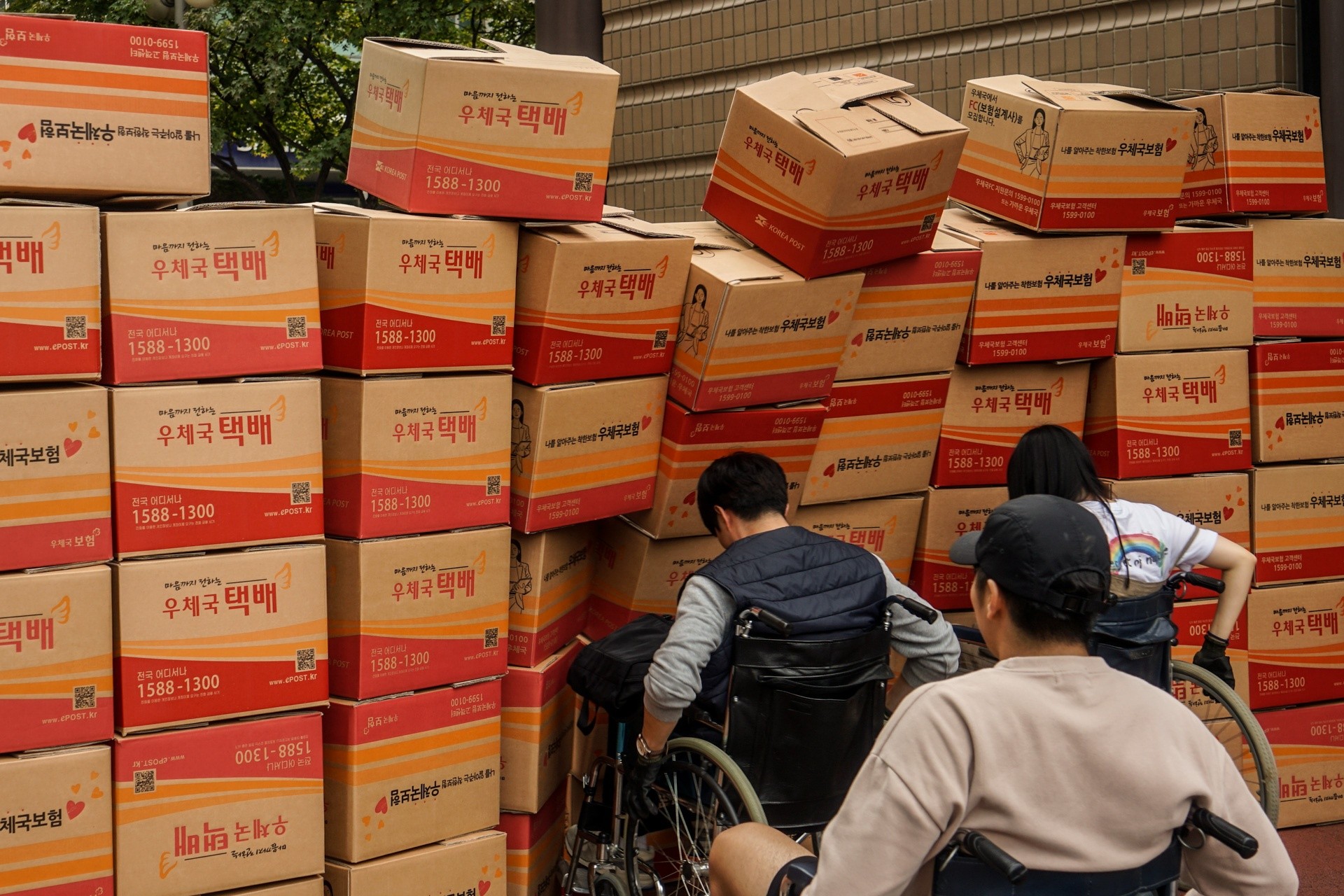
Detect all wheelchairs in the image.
[765,800,1256,896]
[950,569,1279,893]
[558,595,937,896]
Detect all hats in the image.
[949,493,1118,615]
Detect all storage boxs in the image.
[0,12,1344,896]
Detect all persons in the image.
[561,451,962,896]
[1005,425,1259,701]
[708,495,1300,896]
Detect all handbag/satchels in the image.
[565,612,732,737]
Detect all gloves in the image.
[1195,631,1234,704]
[621,757,662,824]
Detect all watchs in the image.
[636,734,670,758]
